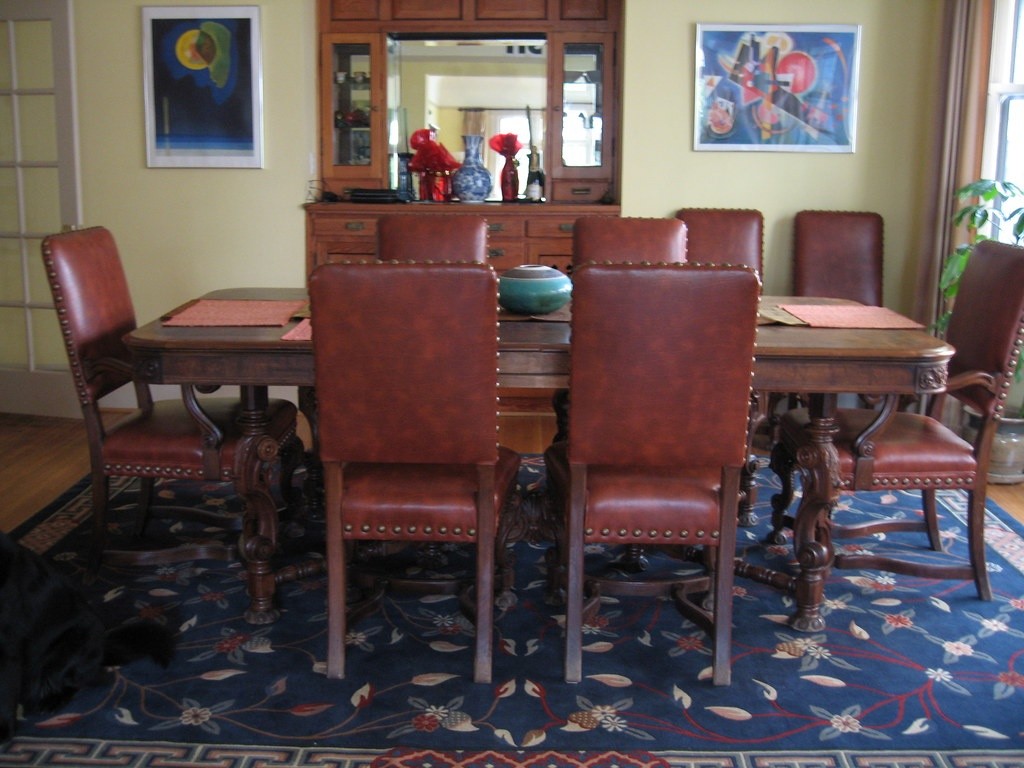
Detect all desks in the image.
[120,288,956,633]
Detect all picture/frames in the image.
[143,7,264,170]
[693,24,861,155]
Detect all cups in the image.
[355,71,364,84]
[336,71,347,83]
[419,170,451,202]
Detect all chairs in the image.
[39,208,1024,688]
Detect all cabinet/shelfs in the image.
[303,214,615,276]
[314,0,621,201]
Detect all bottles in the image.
[498,263,573,315]
[452,135,493,204]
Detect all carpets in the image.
[0,468,1024,768]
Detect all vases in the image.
[928,180,1024,484]
[452,135,492,201]
[501,154,519,201]
